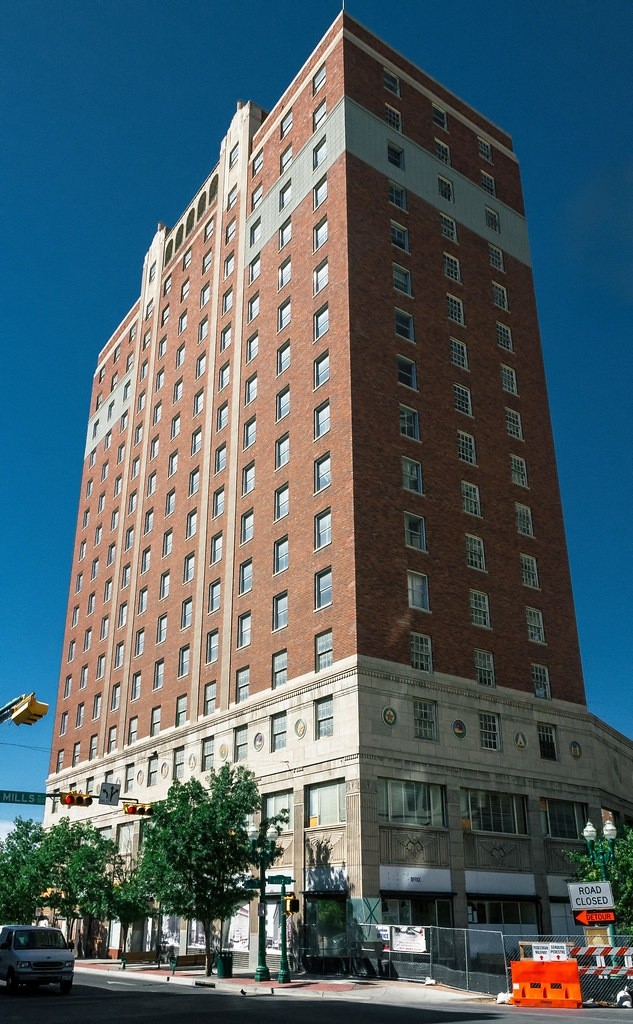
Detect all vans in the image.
[0,924,75,995]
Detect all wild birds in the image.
[240,988,247,997]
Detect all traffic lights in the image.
[10,697,50,726]
[60,793,93,807]
[123,804,156,817]
[287,899,299,913]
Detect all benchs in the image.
[120,951,163,970]
[171,953,216,976]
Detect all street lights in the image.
[584,819,617,965]
[254,824,278,981]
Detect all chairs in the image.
[9,936,21,946]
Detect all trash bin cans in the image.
[217,952,233,978]
